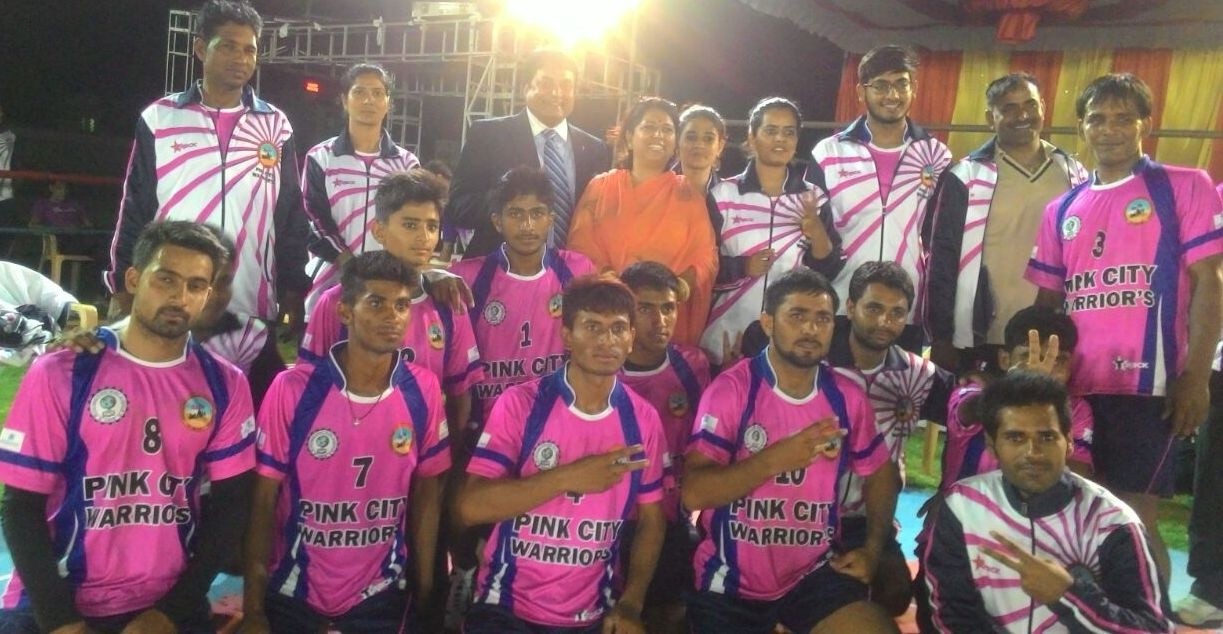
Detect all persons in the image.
[0,1,1223,634]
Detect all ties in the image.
[541,127,575,250]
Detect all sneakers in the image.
[444,563,480,633]
[1170,585,1223,624]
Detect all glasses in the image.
[864,79,913,92]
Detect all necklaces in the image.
[342,383,390,425]
